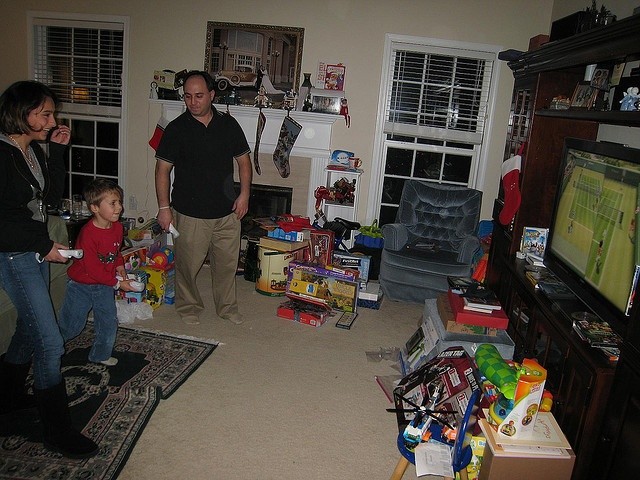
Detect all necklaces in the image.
[8,135,34,169]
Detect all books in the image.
[460,288,502,314]
[520,226,550,259]
[478,407,573,460]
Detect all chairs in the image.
[389,390,481,479]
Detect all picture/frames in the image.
[309,87,345,115]
[204,20,305,110]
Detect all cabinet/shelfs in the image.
[323,164,363,224]
[537,11,638,125]
[487,48,597,306]
[500,253,640,479]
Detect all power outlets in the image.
[129,195,137,210]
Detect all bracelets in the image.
[157,205,171,209]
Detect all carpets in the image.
[3,318,222,480]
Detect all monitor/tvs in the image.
[543,135,640,339]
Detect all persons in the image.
[256,64,264,94]
[154,69,253,326]
[261,67,286,95]
[1,81,100,461]
[59,178,137,366]
[340,98,351,128]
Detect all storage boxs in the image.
[474,396,577,480]
[421,298,516,359]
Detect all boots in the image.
[0,353,38,418]
[31,378,98,459]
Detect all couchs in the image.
[377,179,483,304]
[1,215,75,355]
